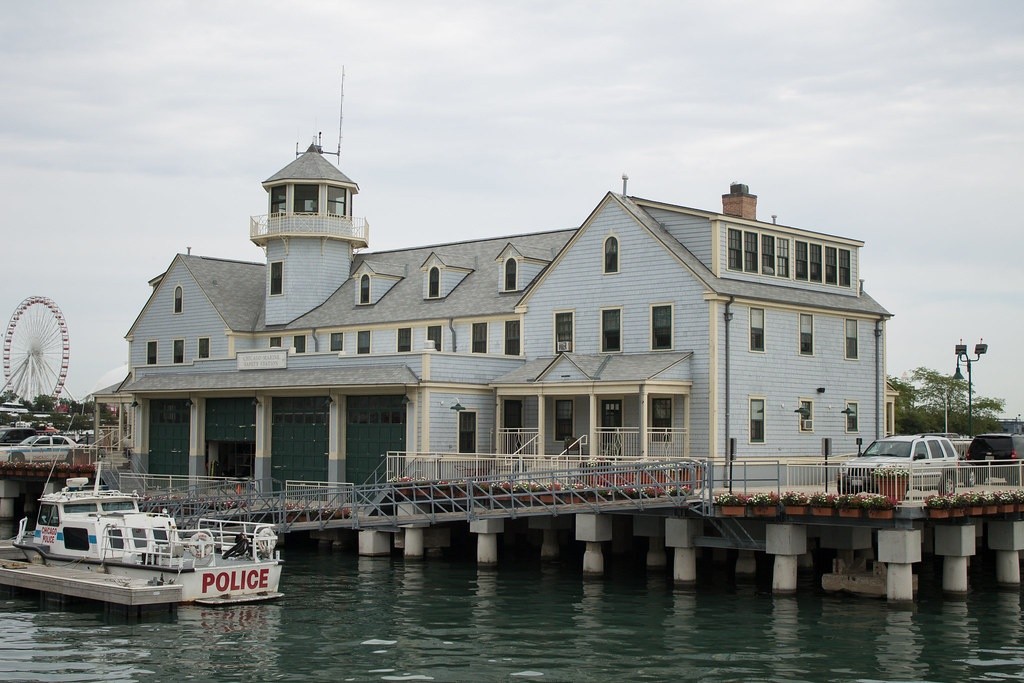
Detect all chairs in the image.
[564,436,584,461]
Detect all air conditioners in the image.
[557,341,572,351]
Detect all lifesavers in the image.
[257,527,278,553]
[188,532,215,558]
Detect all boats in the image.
[10,463,286,605]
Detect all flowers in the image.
[143,493,240,508]
[285,503,351,518]
[388,476,692,499]
[872,466,909,479]
[924,489,1024,509]
[716,491,896,512]
[0,461,96,474]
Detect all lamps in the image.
[780,404,810,414]
[817,388,825,393]
[325,397,333,404]
[251,397,259,406]
[828,405,855,414]
[440,397,466,414]
[129,401,138,407]
[186,399,194,406]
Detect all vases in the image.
[0,470,93,479]
[927,504,1024,518]
[154,507,194,514]
[722,507,893,520]
[397,490,597,504]
[876,476,908,501]
[285,514,307,522]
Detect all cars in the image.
[955,450,977,487]
[0,435,90,465]
[0,427,38,447]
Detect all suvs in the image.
[836,434,960,501]
[965,432,1024,486]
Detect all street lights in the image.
[951,338,988,440]
